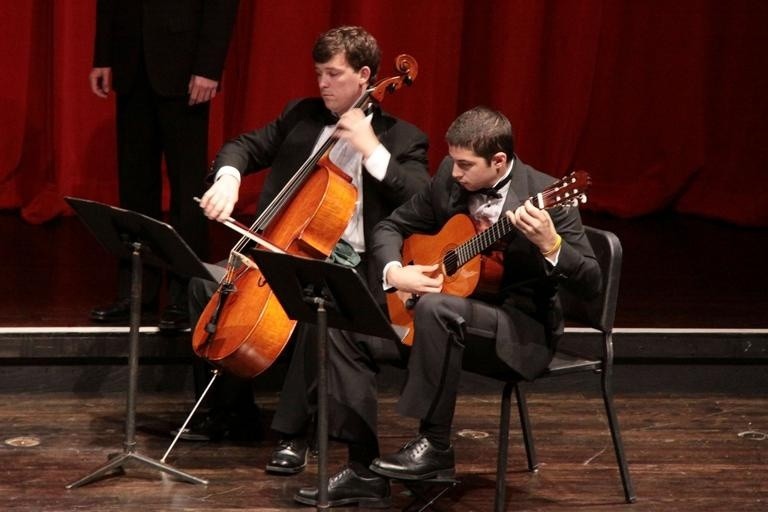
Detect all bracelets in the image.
[540,235,563,258]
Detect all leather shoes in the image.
[158,300,191,328]
[369,437,459,483]
[292,469,393,510]
[263,437,314,473]
[169,407,263,441]
[86,291,149,324]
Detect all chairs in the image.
[448,226,637,512]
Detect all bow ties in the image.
[472,154,517,200]
[321,105,377,126]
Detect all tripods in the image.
[63,244,210,490]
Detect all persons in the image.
[294,104,606,509]
[90,0,240,329]
[169,25,429,475]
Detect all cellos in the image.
[191,53,419,380]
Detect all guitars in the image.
[385,168,592,348]
